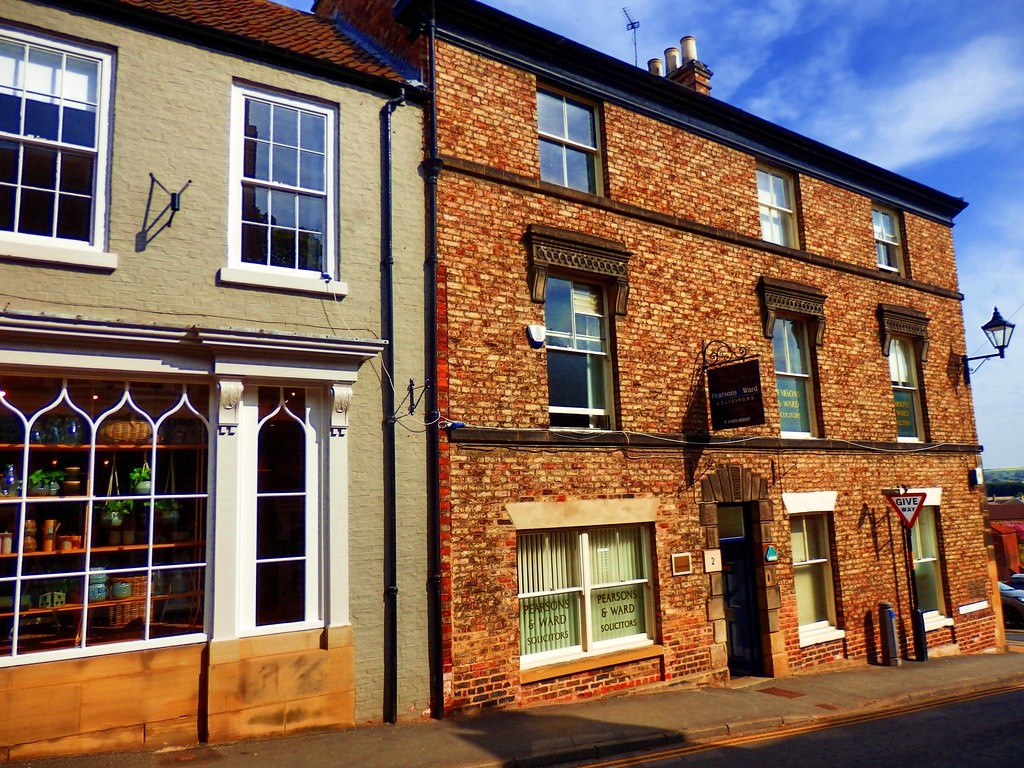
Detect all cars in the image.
[998,581,1024,627]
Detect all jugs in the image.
[30,417,83,444]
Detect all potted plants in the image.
[27,470,68,497]
[92,500,135,528]
[144,500,180,523]
[128,467,151,494]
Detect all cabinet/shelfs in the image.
[0,421,208,657]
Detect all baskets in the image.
[96,418,165,446]
[97,575,154,628]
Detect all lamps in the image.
[150,173,191,227]
[960,306,1016,384]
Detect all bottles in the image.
[89,567,132,603]
[154,562,166,596]
[108,515,136,545]
[171,562,190,593]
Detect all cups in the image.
[0,518,81,554]
